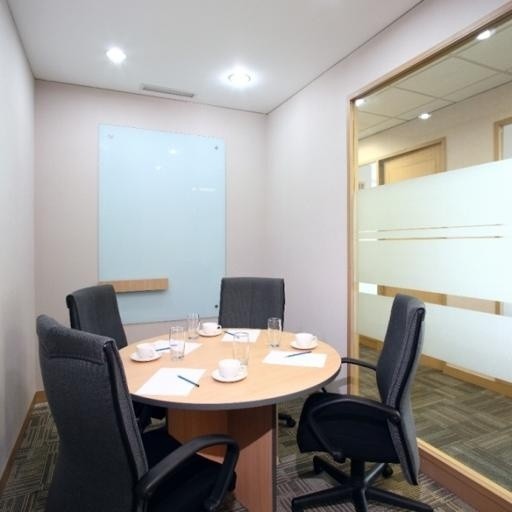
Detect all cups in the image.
[202,321,222,334]
[267,316,281,348]
[295,333,317,347]
[232,332,249,366]
[136,342,156,359]
[186,310,201,341]
[218,359,246,379]
[169,326,186,362]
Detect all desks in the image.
[117,327,341,511]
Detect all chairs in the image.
[292,294,436,512]
[219,277,285,329]
[37,315,240,512]
[66,285,127,351]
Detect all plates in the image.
[130,350,161,362]
[290,340,317,350]
[199,329,220,337]
[212,364,248,383]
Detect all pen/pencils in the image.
[177,376,199,387]
[224,330,234,336]
[288,350,312,357]
[156,347,170,352]
